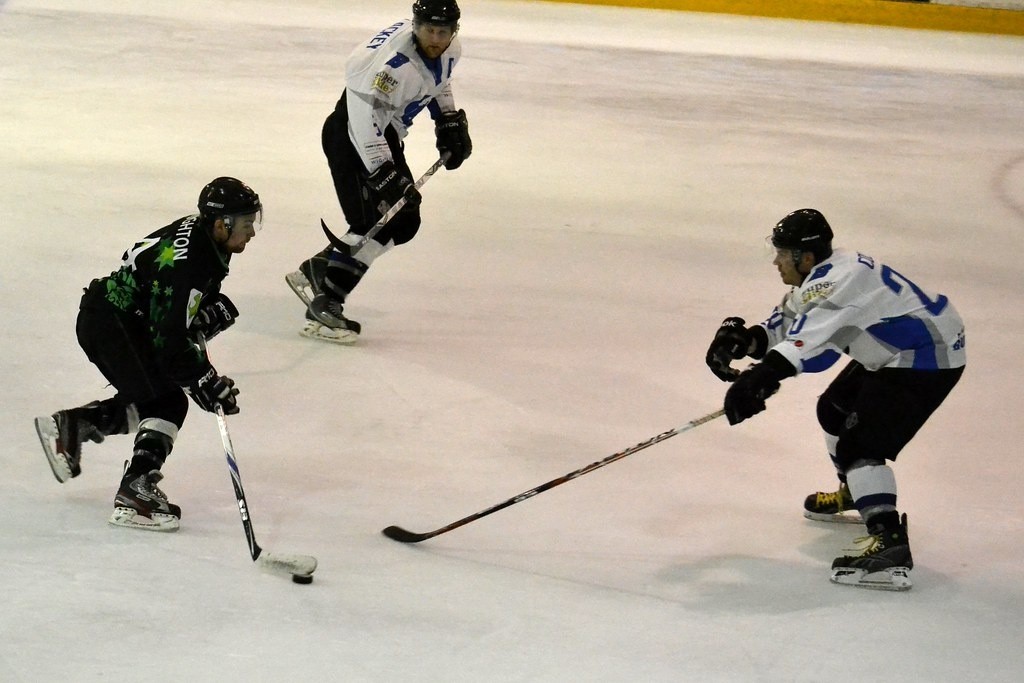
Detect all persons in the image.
[286,0,473,343]
[35,176,262,531]
[706,209,967,592]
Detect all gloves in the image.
[186,359,240,416]
[367,161,421,226]
[706,317,753,382]
[435,109,472,170]
[724,350,798,426]
[188,293,239,344]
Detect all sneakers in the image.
[299,292,361,346]
[285,255,336,308]
[830,512,914,592]
[108,459,182,533]
[803,472,866,525]
[34,408,105,484]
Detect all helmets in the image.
[412,0,460,27]
[197,176,262,218]
[771,209,833,250]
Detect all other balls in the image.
[292,573,312,585]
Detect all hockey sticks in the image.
[198,331,317,578]
[314,151,453,257]
[382,409,724,549]
